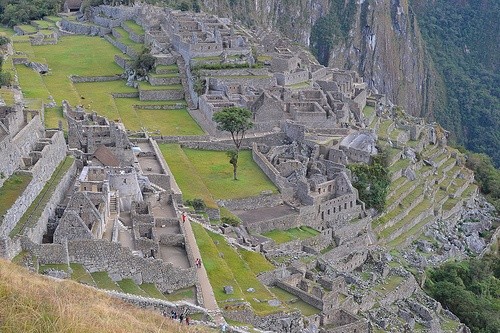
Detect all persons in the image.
[183,215,186,223]
[163,309,191,325]
[198,259,202,267]
[196,258,199,267]
